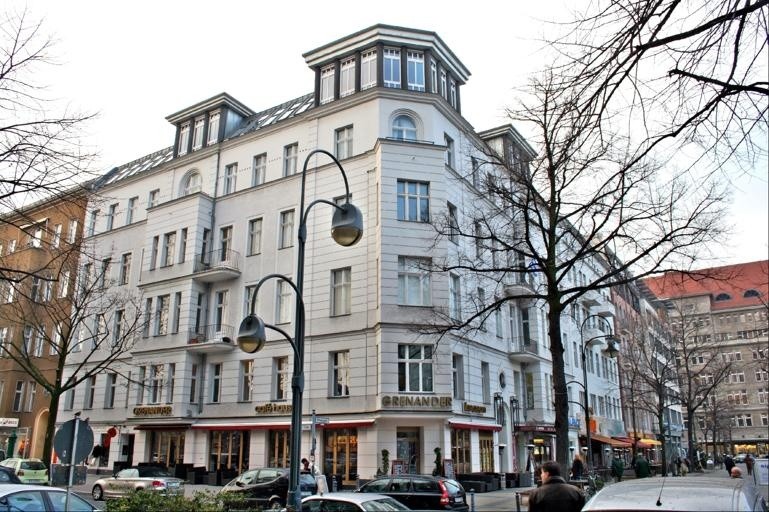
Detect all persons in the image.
[725,455,735,476]
[528,462,586,512]
[674,456,682,475]
[631,452,652,478]
[572,453,584,479]
[615,458,624,482]
[745,454,753,475]
[301,458,321,475]
[682,458,692,473]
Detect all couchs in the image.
[456,472,531,492]
[113,461,238,486]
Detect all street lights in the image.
[234,148,366,511]
[581,314,620,494]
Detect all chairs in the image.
[336,474,356,491]
